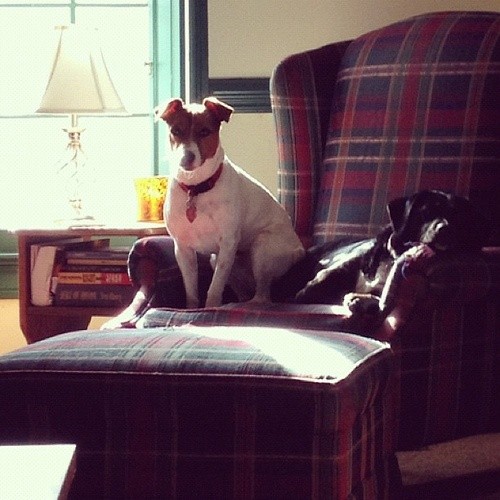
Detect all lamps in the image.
[36,26,127,223]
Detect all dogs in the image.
[151,95,306,310]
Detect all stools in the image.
[0,324,395,499]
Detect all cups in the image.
[137,177,168,222]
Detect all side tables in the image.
[15,226,169,344]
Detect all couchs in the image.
[101,11,500,453]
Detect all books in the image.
[52,249,133,307]
